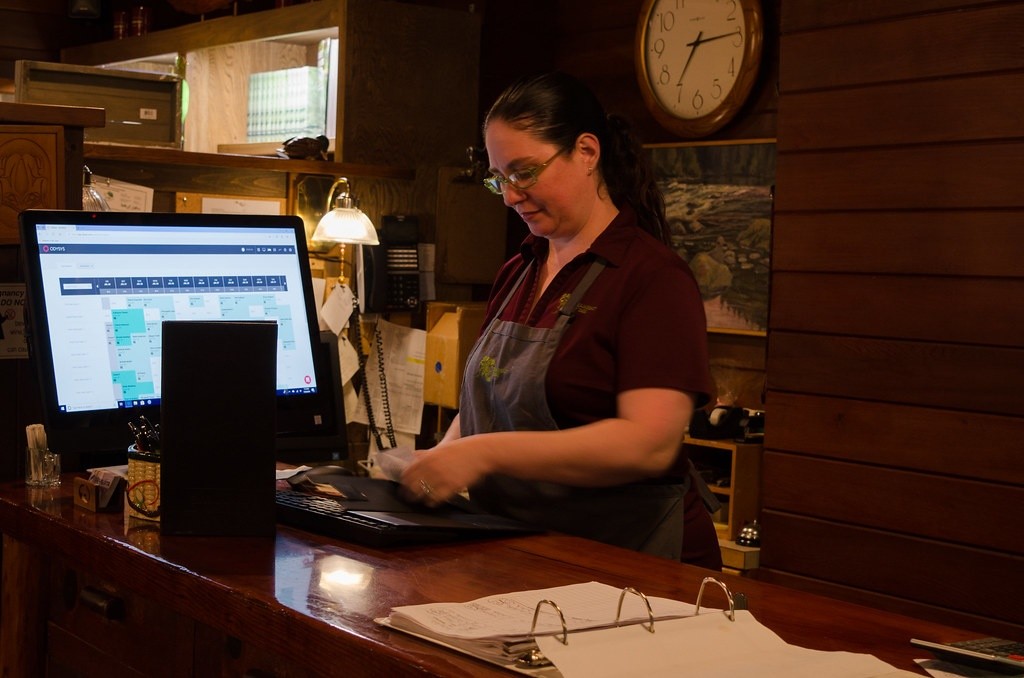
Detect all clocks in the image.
[634,0,765,142]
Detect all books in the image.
[371,580,940,678]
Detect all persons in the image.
[378,70,724,572]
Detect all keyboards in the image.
[275,489,422,549]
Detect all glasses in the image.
[481,146,566,194]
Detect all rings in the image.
[418,480,434,495]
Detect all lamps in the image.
[311,177,382,248]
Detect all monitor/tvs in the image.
[20,208,335,455]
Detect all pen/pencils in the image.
[127,413,160,452]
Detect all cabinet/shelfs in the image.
[681,435,763,540]
